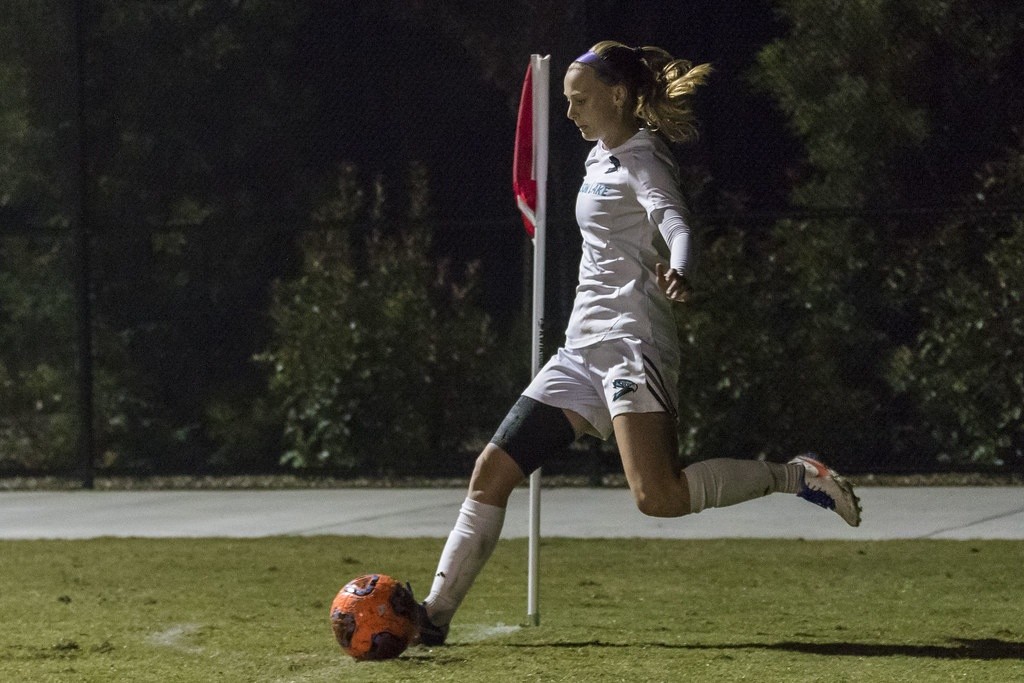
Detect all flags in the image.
[513,63,536,240]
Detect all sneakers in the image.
[406,579,448,644]
[789,451,863,529]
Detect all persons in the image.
[415,41,863,646]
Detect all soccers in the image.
[329,573,413,661]
